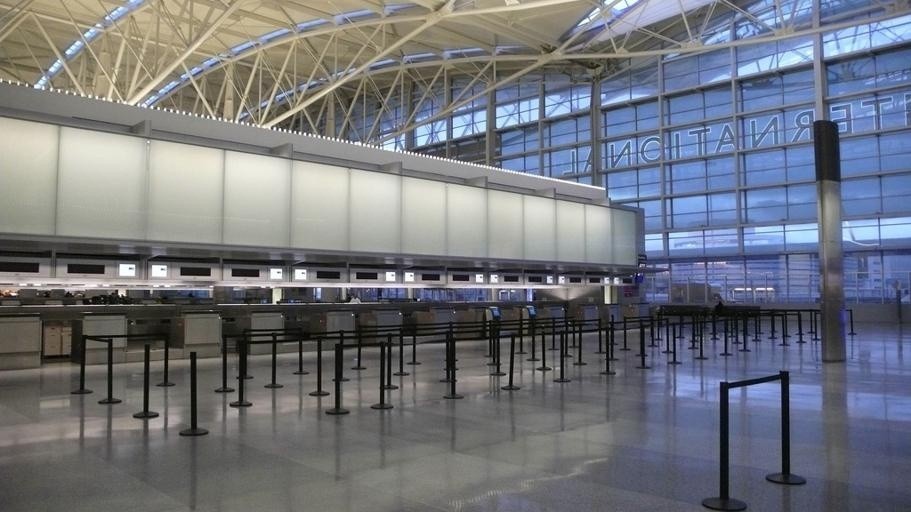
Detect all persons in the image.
[713,296,724,307]
[349,292,361,303]
